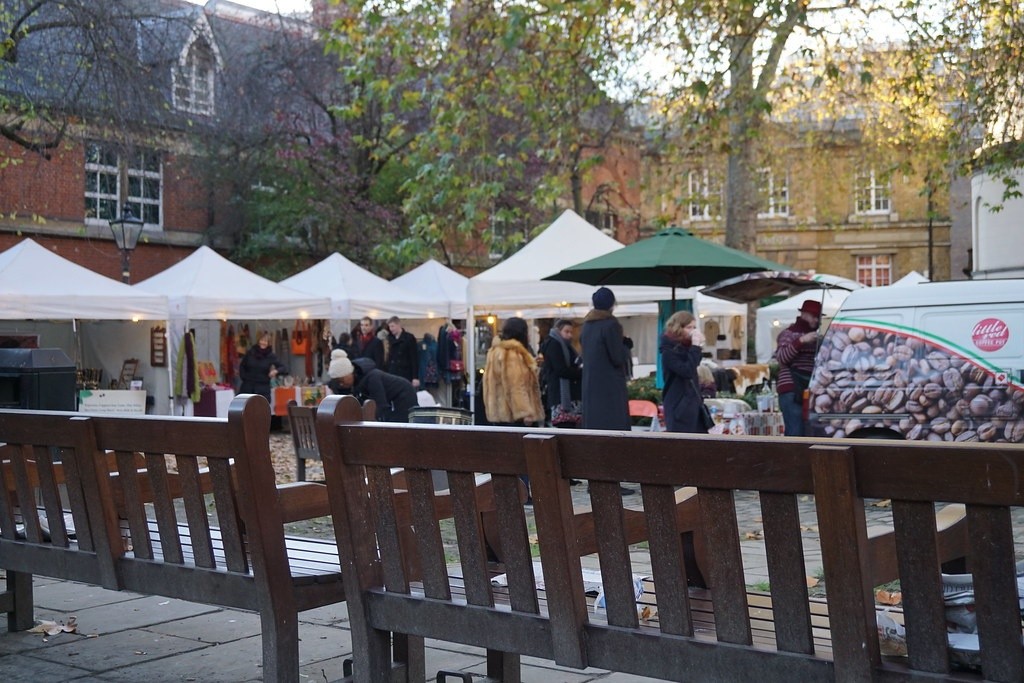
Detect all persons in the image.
[238,287,826,504]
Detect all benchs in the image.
[0,394,424,683]
[317,397,1024,683]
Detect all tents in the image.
[0,208,931,425]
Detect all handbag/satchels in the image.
[701,402,715,431]
[790,367,812,405]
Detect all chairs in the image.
[629,400,660,432]
[286,400,376,481]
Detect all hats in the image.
[327,349,354,378]
[592,287,615,309]
[798,300,827,316]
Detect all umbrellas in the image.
[540,226,800,314]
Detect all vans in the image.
[808,276,1024,443]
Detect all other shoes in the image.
[523,495,534,505]
[620,486,635,495]
[570,480,582,486]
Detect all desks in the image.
[709,411,788,436]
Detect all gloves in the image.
[623,336,634,349]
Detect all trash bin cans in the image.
[406,405,474,491]
[0,347,78,463]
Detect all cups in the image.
[689,329,705,346]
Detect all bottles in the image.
[762,381,770,412]
[771,381,779,411]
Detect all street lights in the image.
[108,201,144,287]
